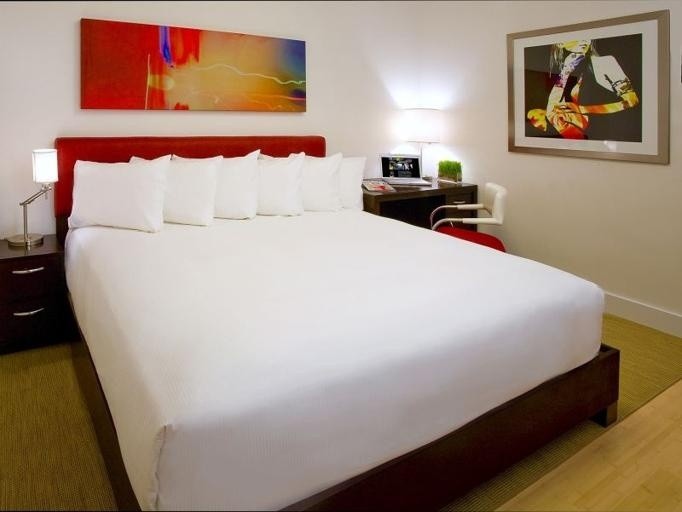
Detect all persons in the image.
[526,39,639,139]
[402,163,406,171]
[393,160,399,177]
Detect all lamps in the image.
[5,148,59,249]
[401,108,446,181]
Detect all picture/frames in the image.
[504,7,670,167]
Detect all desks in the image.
[359,172,477,229]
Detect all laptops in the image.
[381,155,432,185]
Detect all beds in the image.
[52,135,619,511]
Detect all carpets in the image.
[1,308,682,511]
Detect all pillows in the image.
[290,153,341,211]
[68,156,170,233]
[173,151,259,222]
[130,154,222,227]
[253,151,306,217]
[331,156,368,210]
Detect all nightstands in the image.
[1,233,77,355]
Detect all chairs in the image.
[428,183,509,254]
